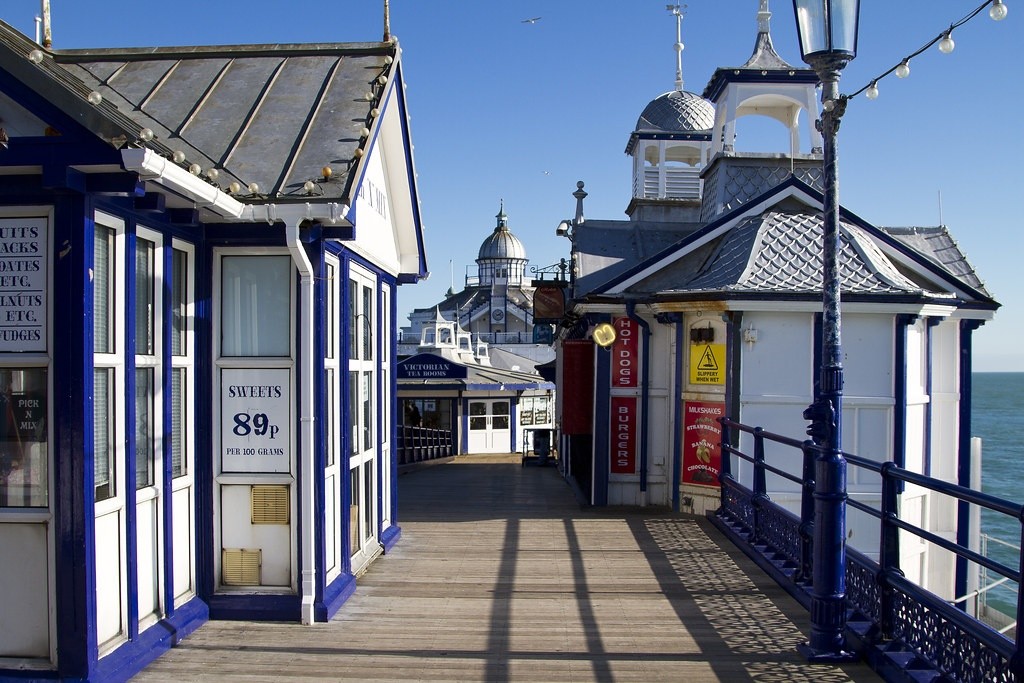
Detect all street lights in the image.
[791,0,861,664]
